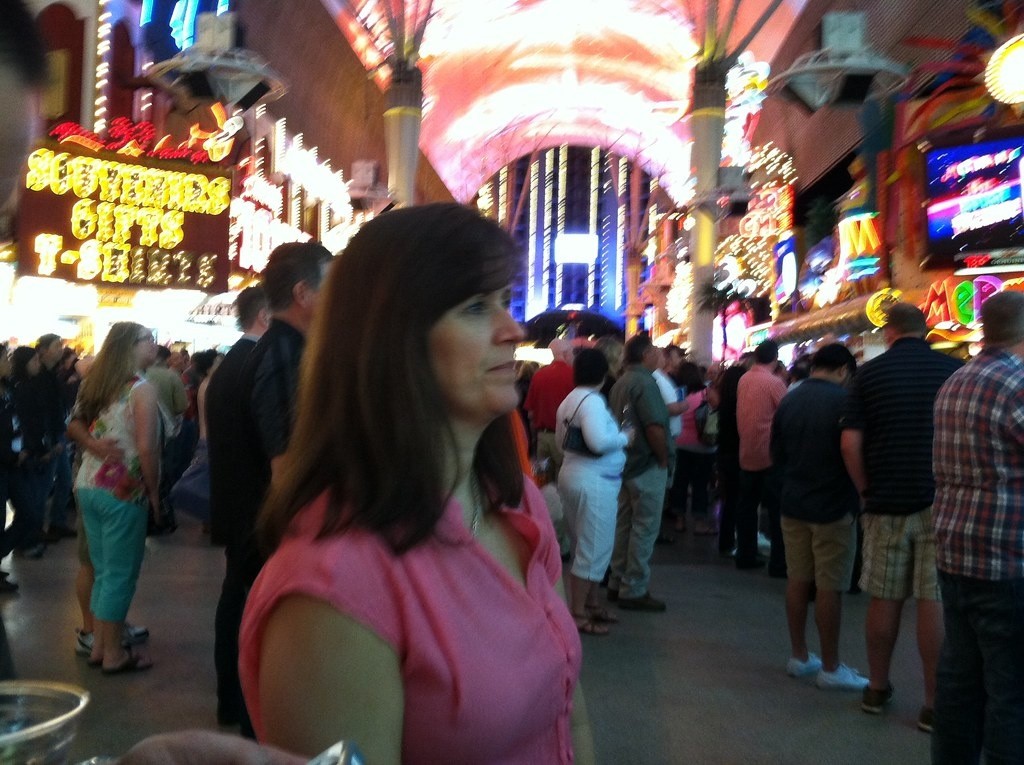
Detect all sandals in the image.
[576,606,616,635]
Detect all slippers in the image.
[675,526,687,532]
[693,527,719,536]
[86,653,153,675]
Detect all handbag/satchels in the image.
[168,439,210,521]
[694,403,718,446]
[560,392,608,458]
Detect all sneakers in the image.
[816,663,869,691]
[861,681,895,713]
[918,705,933,732]
[787,652,822,677]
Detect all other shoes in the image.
[606,588,666,612]
[737,559,765,570]
[77,626,150,650]
[14,545,46,559]
[768,565,787,578]
[0,572,19,593]
[47,524,78,539]
[719,542,738,557]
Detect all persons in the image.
[838,303,965,732]
[233,243,334,743]
[237,201,593,764]
[517,330,814,635]
[0,0,55,765]
[929,292,1024,765]
[205,284,272,742]
[64,322,159,674]
[0,333,225,655]
[770,345,869,690]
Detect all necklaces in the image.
[472,475,481,538]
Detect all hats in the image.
[15,346,37,365]
